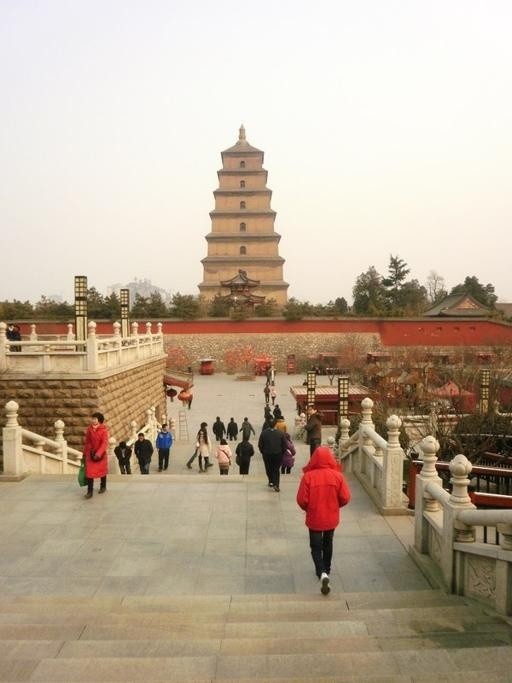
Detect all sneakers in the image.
[320,573,330,595]
[86,492,92,498]
[269,482,280,491]
[99,487,106,493]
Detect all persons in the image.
[5,324,14,352]
[12,326,21,352]
[83,412,109,500]
[115,366,324,492]
[388,384,414,410]
[296,444,352,596]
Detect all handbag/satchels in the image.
[78,459,88,486]
[90,448,104,461]
